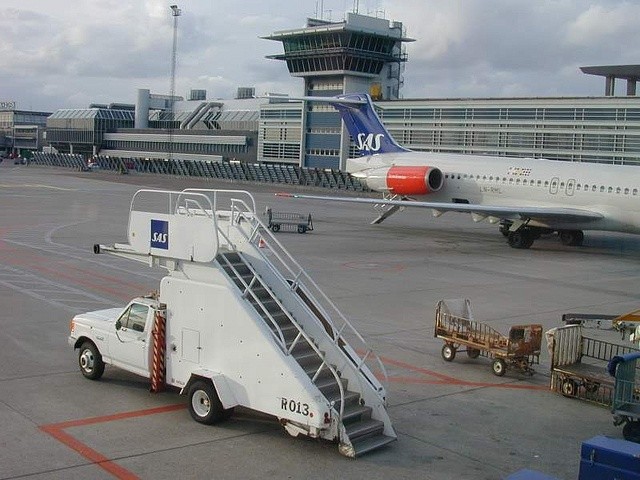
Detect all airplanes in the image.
[252,90,640,250]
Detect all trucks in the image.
[434,297,544,377]
[610,347,640,443]
[263,205,314,234]
[548,323,640,411]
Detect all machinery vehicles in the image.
[66,185,401,460]
